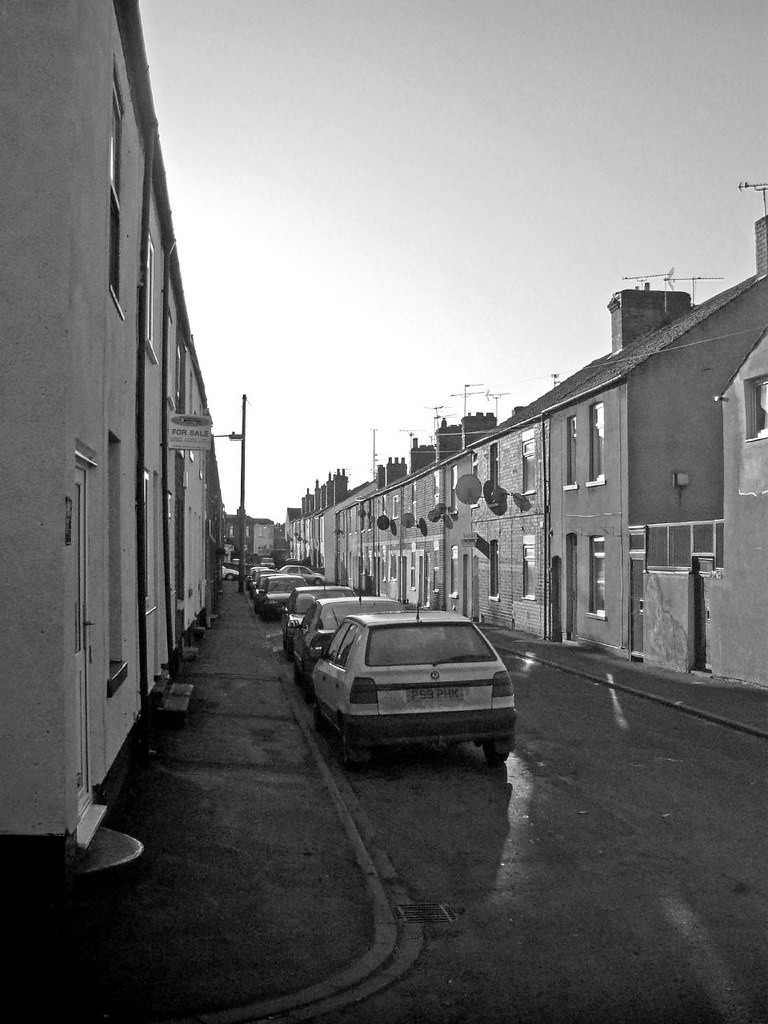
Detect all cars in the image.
[244,567,289,599]
[280,586,357,660]
[232,559,240,564]
[311,611,516,768]
[279,565,327,586]
[254,576,308,620]
[286,596,407,701]
[261,558,275,569]
[222,566,239,581]
[285,558,302,564]
